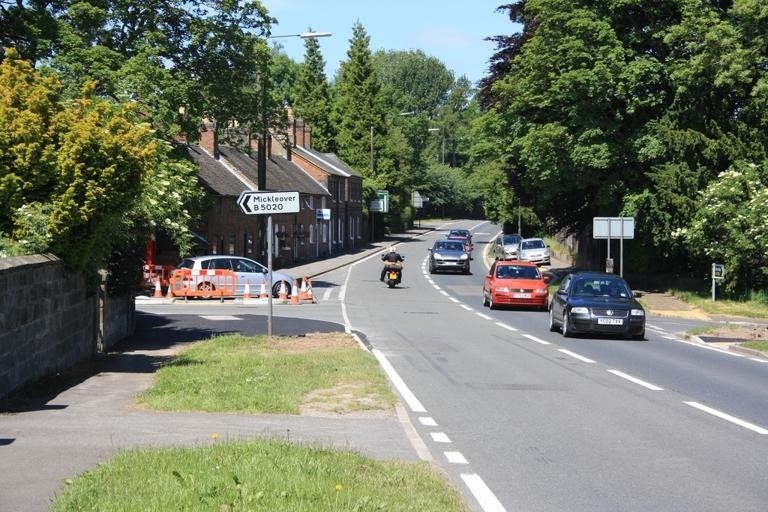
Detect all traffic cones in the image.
[164,280,175,300]
[241,272,315,305]
[152,275,163,298]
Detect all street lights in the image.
[255,25,333,267]
[368,110,414,177]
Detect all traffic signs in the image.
[234,190,303,215]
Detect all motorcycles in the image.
[379,253,406,287]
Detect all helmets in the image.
[389,245,396,253]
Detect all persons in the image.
[380,246,404,281]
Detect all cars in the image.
[168,255,297,299]
[426,239,471,275]
[479,259,553,313]
[545,270,649,345]
[493,233,525,262]
[445,226,473,262]
[514,238,553,267]
